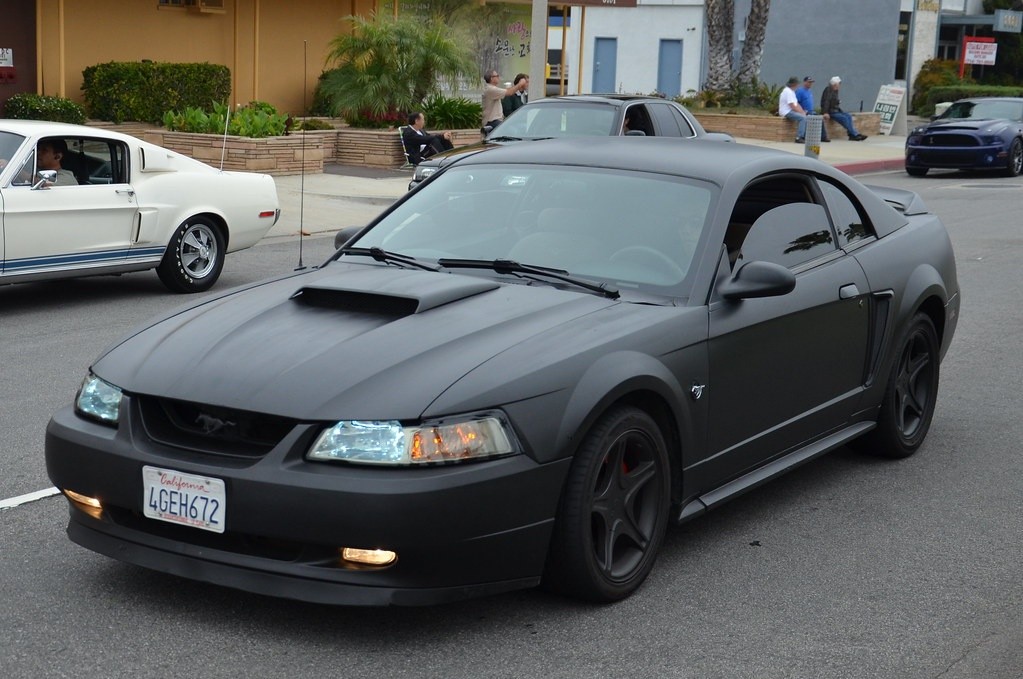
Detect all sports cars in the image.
[904,97,1023,177]
[46,137,963,610]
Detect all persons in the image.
[796,75,831,143]
[821,76,868,141]
[0,136,79,186]
[483,68,527,132]
[502,73,529,117]
[402,112,454,165]
[778,77,807,143]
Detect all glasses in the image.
[492,75,499,78]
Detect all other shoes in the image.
[796,137,804,143]
[849,135,862,141]
[857,133,868,141]
[822,138,831,143]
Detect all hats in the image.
[803,76,815,83]
[785,77,801,86]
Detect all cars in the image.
[0,120,282,294]
[405,93,736,196]
